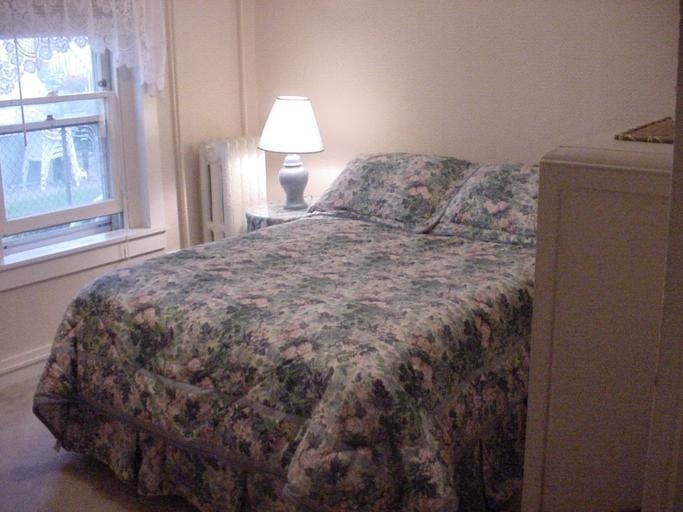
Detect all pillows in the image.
[308,150,540,245]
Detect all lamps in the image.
[256,95,325,210]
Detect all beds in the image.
[65,211,536,511]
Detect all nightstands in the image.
[244,205,311,235]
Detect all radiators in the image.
[196,134,266,245]
